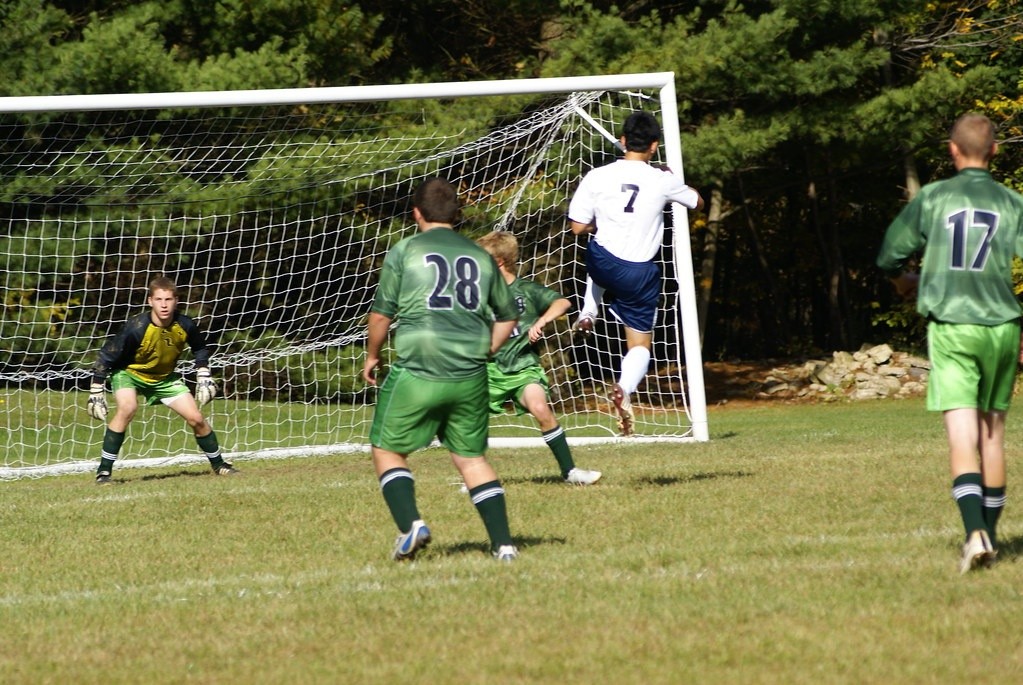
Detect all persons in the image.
[878,113,1023,576]
[362,178,520,562]
[87,278,241,486]
[568,111,704,436]
[473,231,602,486]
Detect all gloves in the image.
[86,383,113,421]
[194,367,219,406]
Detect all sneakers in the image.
[95,470,112,483]
[214,460,240,476]
[959,528,998,576]
[563,467,602,487]
[391,519,433,562]
[571,312,596,340]
[607,383,636,436]
[490,544,520,562]
